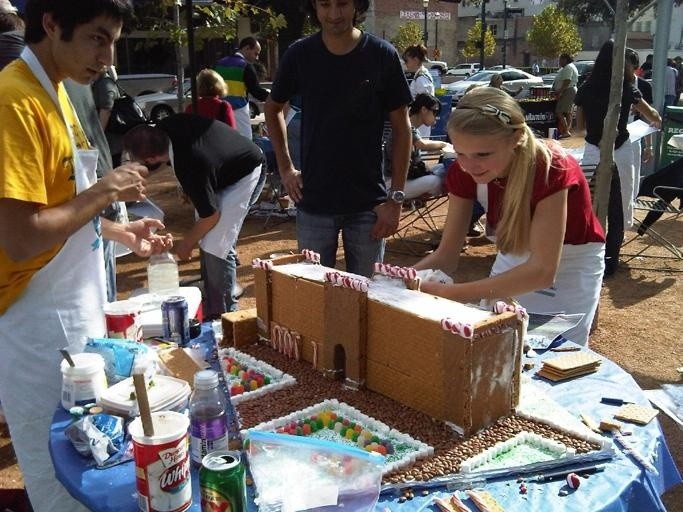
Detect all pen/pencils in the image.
[152,338,170,345]
[551,337,581,352]
[537,465,606,482]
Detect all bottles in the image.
[147,244,179,302]
[189,370,228,469]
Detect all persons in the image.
[1,1,272,510]
[412,87,606,349]
[552,39,683,277]
[264,1,414,276]
[383,44,538,238]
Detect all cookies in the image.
[536,351,602,382]
[600,421,621,431]
[580,413,603,434]
[432,491,506,512]
[615,404,659,425]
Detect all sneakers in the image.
[559,132,571,138]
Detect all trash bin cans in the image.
[430,96,451,141]
[660,105,683,170]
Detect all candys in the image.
[251,410,394,474]
[218,355,271,396]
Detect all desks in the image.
[47,299,682,512]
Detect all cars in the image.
[398,53,448,78]
[446,62,484,78]
[442,67,543,106]
[541,60,595,87]
[134,77,271,124]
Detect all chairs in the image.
[614,185,682,269]
[413,137,479,230]
[380,141,449,253]
[252,111,307,230]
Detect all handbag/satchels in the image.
[96,76,148,140]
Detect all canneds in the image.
[161,296,190,347]
[199,450,248,512]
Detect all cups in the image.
[103,300,144,343]
[128,411,192,512]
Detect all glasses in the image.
[427,107,440,117]
